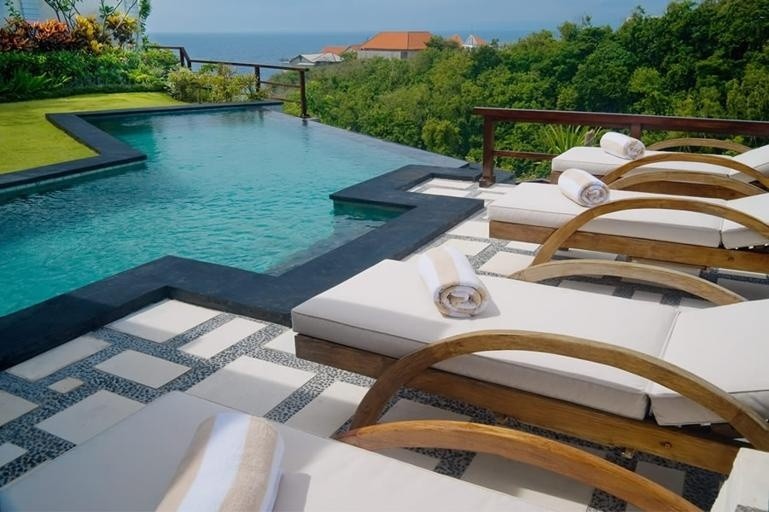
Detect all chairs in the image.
[474,170,769,308]
[285,248,769,486]
[0,384,769,512]
[546,140,768,200]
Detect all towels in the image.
[600,131,645,160]
